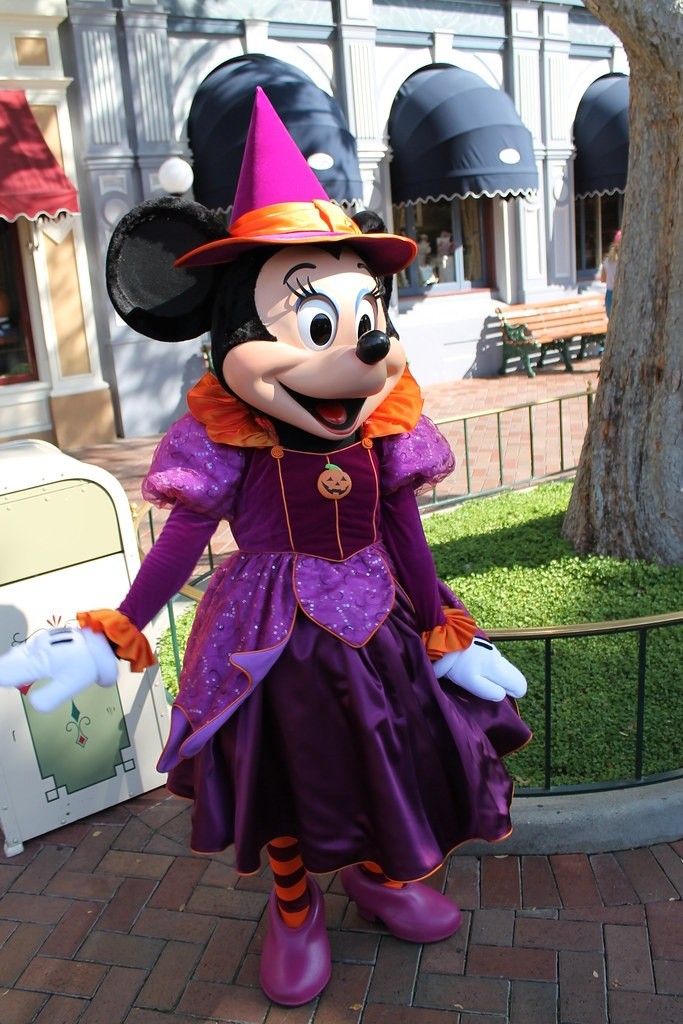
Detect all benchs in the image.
[495,294,608,379]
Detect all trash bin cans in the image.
[0,437,166,858]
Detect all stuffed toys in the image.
[0,87,532,1006]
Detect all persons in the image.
[600,230,621,317]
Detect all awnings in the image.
[388,63,538,208]
[0,90,81,223]
[573,72,630,199]
[187,54,363,215]
